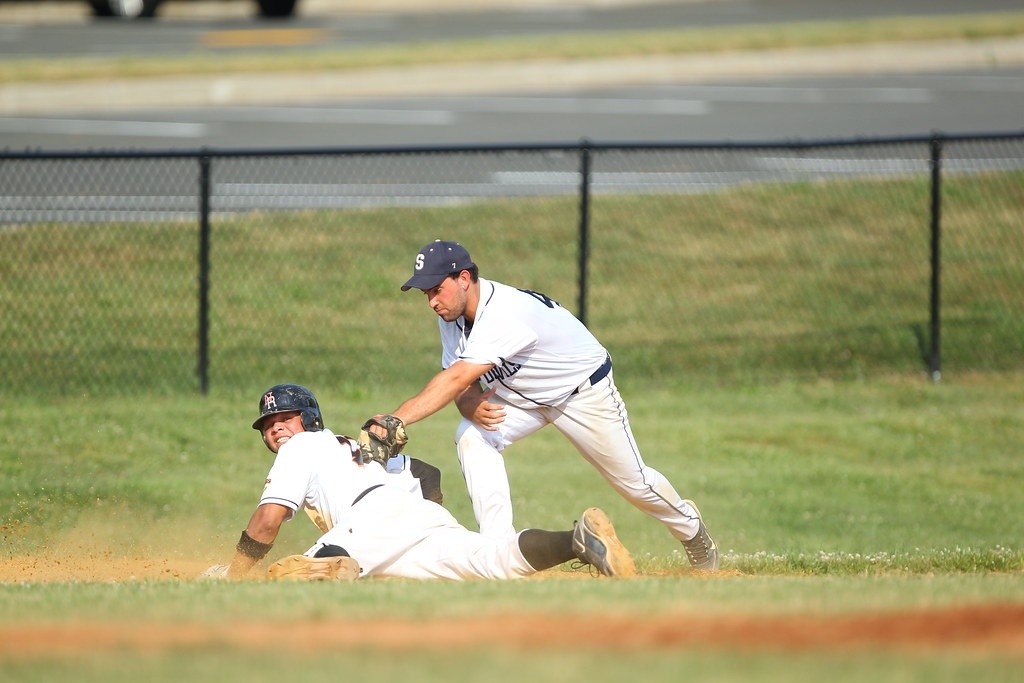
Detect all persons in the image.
[194,384,636,583]
[359,239,717,572]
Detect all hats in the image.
[401,240,475,292]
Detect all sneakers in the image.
[572,506,634,578]
[266,555,361,582]
[681,498,720,572]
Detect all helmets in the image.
[252,383,325,454]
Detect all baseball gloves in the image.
[356,414,408,469]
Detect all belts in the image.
[350,484,385,506]
[572,355,613,396]
